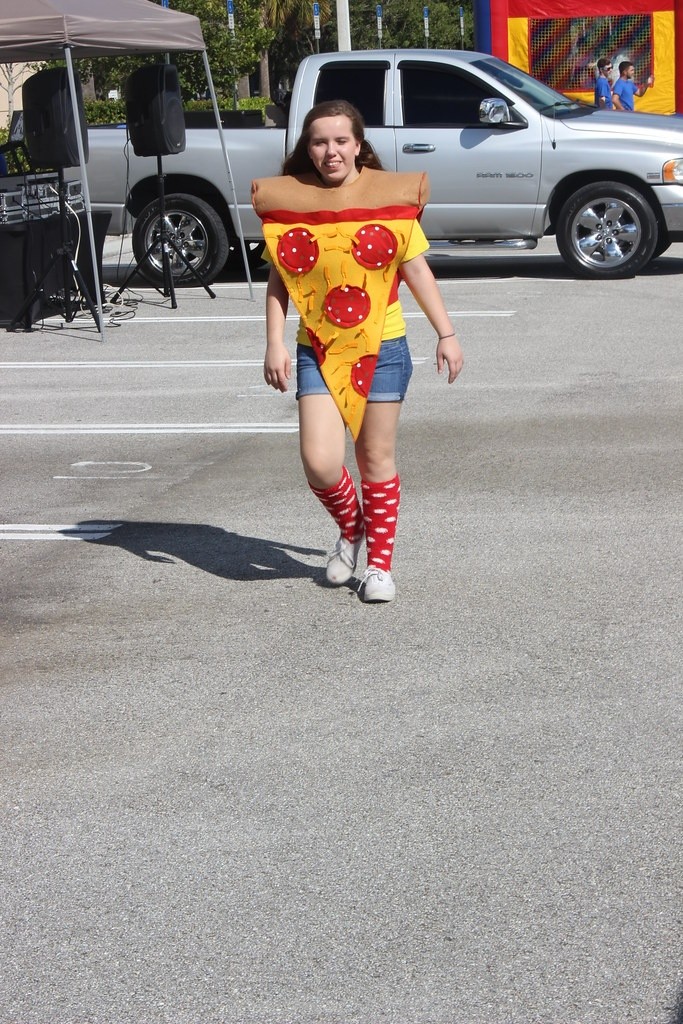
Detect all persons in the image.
[251,100,464,603]
[594,57,654,112]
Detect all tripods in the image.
[110,156,216,310]
[7,169,103,334]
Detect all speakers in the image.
[125,64,185,157]
[23,66,89,170]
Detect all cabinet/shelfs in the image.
[184,110,262,129]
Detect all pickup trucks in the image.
[41,48,683,288]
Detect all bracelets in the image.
[439,333,455,339]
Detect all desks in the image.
[0,210,113,328]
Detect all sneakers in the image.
[325,530,365,585]
[354,567,396,602]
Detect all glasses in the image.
[603,65,613,71]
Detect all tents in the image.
[472,0,683,117]
[0,0,255,333]
